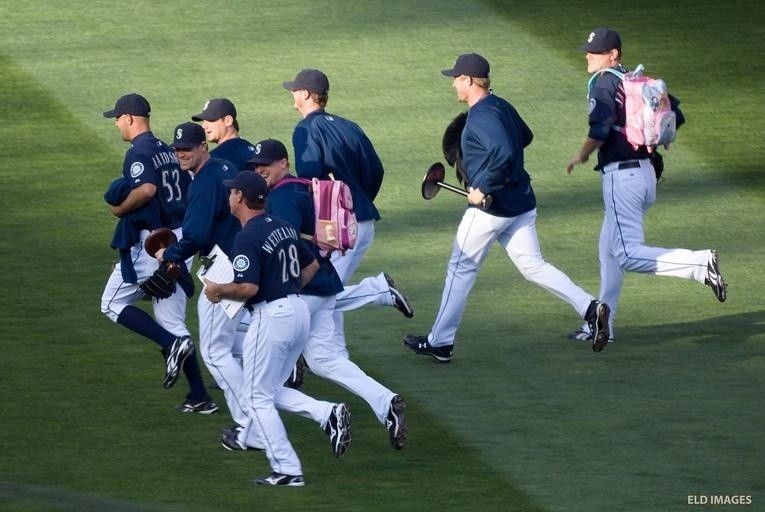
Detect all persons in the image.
[102,68,413,487]
[404,54,609,361]
[567,28,726,343]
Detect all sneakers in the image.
[404,334,454,362]
[288,358,305,390]
[386,395,408,450]
[382,273,413,318]
[704,250,727,302]
[568,300,614,352]
[326,403,352,458]
[222,427,247,452]
[161,336,194,389]
[255,472,306,487]
[175,397,219,414]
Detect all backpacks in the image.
[272,177,358,252]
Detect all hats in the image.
[582,28,622,53]
[192,99,236,121]
[441,53,490,78]
[103,93,151,118]
[283,69,329,94]
[222,170,268,201]
[169,121,207,148]
[247,139,288,166]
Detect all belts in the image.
[249,294,290,313]
[602,161,641,170]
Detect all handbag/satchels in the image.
[622,69,676,146]
